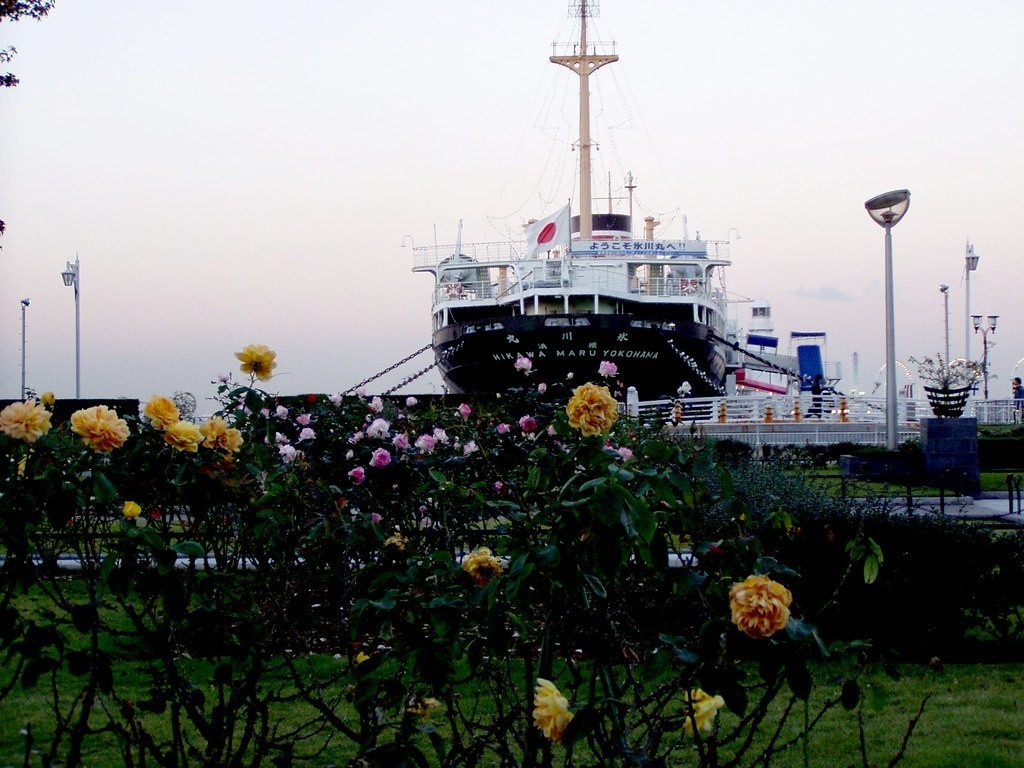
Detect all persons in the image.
[803,374,828,418]
[1013,378,1024,425]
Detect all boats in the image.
[410,0,838,425]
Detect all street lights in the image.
[60,248,82,399]
[864,189,912,451]
[970,315,1000,401]
[939,284,949,372]
[962,237,980,360]
[20,298,32,399]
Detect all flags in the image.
[524,202,572,257]
